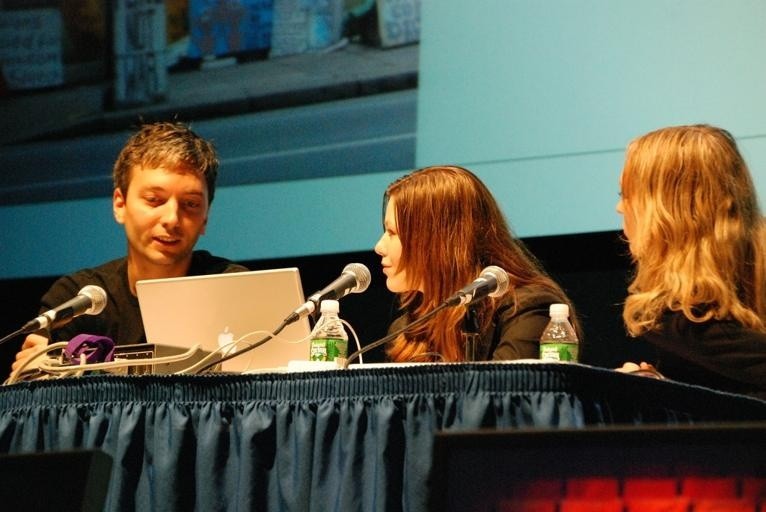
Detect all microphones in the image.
[284,261,371,327]
[22,284,107,333]
[445,264,510,308]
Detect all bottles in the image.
[307,300,348,365]
[538,304,579,363]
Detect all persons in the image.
[376,165,581,361]
[613,124,765,398]
[12,121,248,370]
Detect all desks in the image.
[1,355,766,511]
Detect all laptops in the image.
[135,266,312,371]
